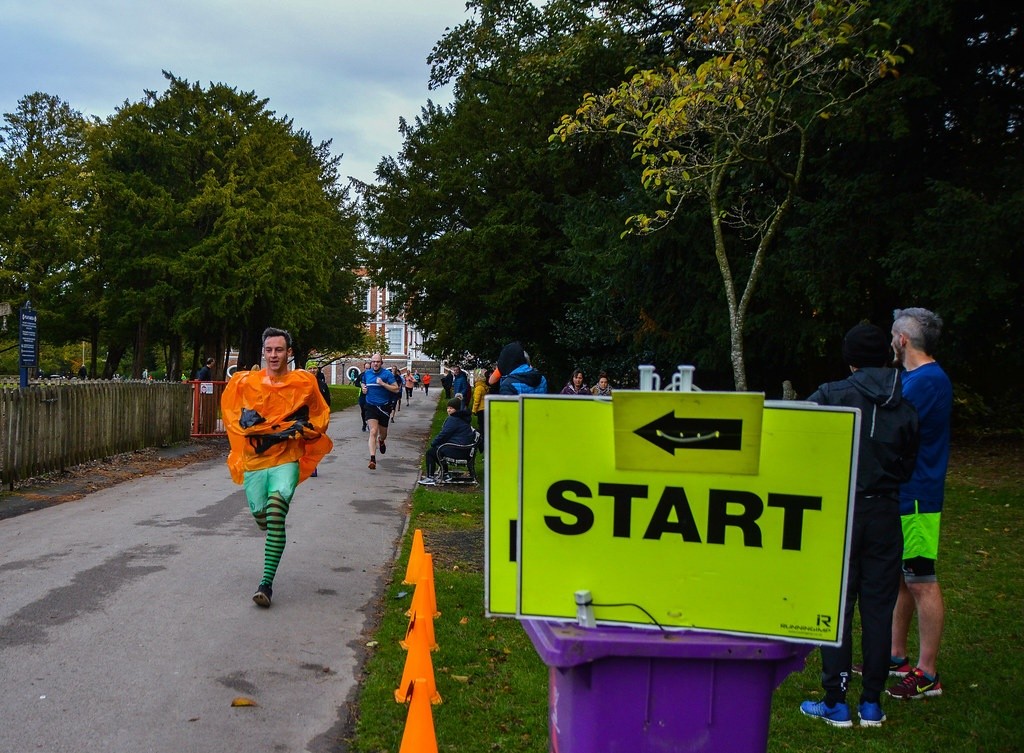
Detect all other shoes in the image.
[362,425,366,432]
[390,417,394,423]
[377,435,386,454]
[253,584,272,607]
[368,461,376,470]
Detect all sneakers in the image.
[885,668,942,698]
[435,473,452,482]
[851,657,911,677]
[417,478,435,484]
[799,697,852,727]
[858,701,887,727]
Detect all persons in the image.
[851,308,953,699]
[77,364,87,380]
[799,319,920,729]
[143,368,152,381]
[182,342,613,487]
[220,326,330,607]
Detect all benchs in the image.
[434,426,480,486]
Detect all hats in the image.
[843,324,890,369]
[305,359,318,371]
[447,393,463,411]
